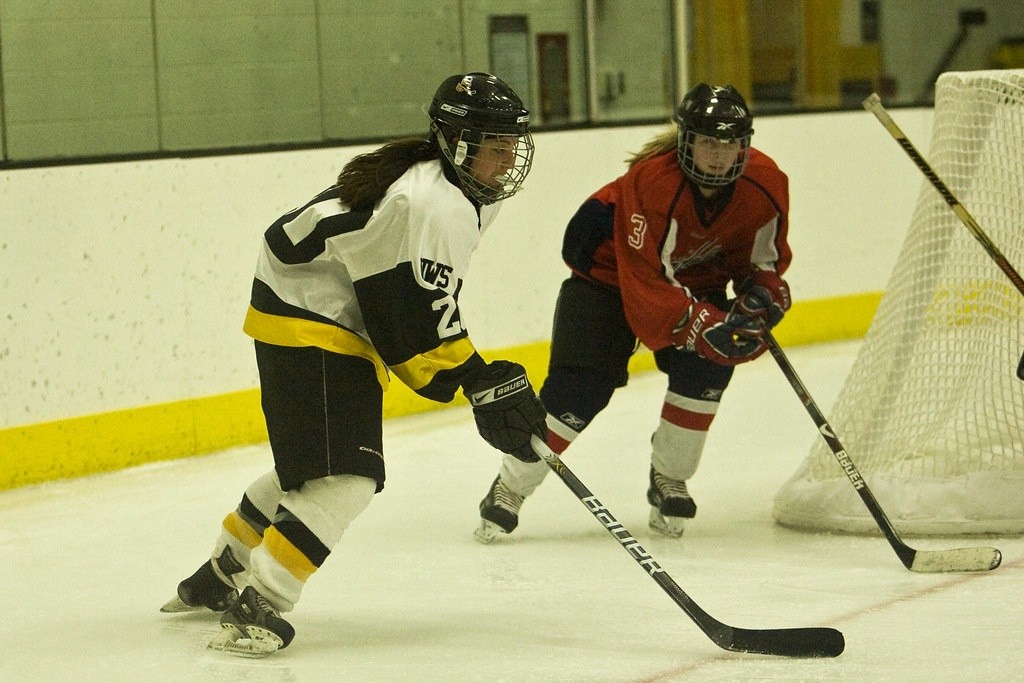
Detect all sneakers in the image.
[647,432,696,538]
[208,586,295,659]
[473,473,524,544]
[159,544,240,612]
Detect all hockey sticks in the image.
[758,325,1003,575]
[529,430,846,661]
[860,91,1024,299]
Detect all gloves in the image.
[463,361,548,463]
[669,302,770,366]
[730,271,791,330]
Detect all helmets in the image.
[672,83,754,186]
[428,72,535,206]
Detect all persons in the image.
[473,83,792,545]
[159,73,535,659]
